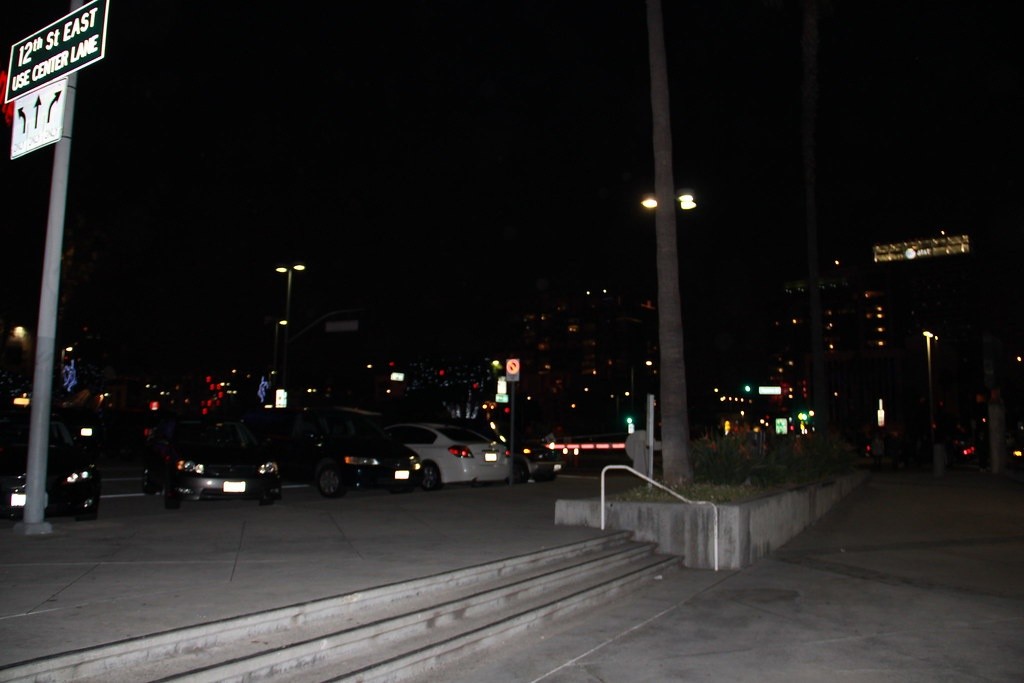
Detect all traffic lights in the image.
[627,418,632,423]
[802,414,808,420]
[744,385,751,392]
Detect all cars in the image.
[506,443,568,485]
[264,407,423,499]
[142,416,284,510]
[382,421,511,492]
[1,417,103,522]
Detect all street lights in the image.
[924,331,935,443]
[276,262,307,387]
